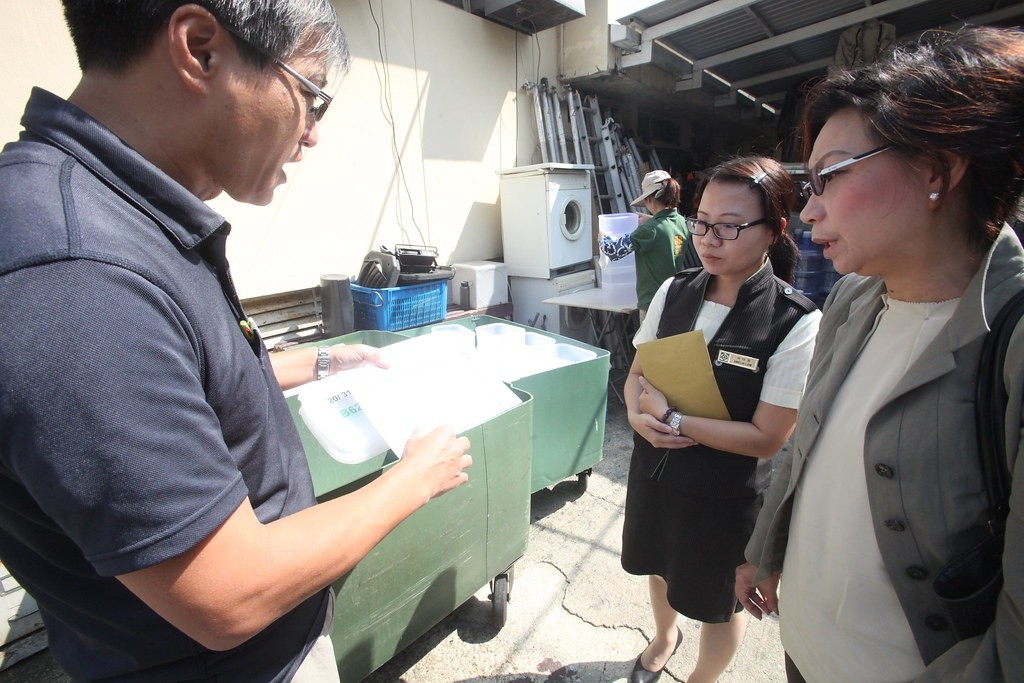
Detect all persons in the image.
[1,0,473,683]
[621,158,822,683]
[734,23,1024,683]
[597,169,690,330]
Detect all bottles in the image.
[461,281,473,309]
[793,231,822,301]
[794,229,803,247]
[821,259,844,301]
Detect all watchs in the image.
[670,412,683,437]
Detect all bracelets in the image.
[660,406,678,423]
[317,345,329,380]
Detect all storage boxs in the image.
[350,279,448,331]
[597,213,639,307]
[270,314,611,683]
[451,261,508,309]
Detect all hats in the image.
[630,170,671,206]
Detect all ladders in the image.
[524,83,641,369]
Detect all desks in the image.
[542,288,639,404]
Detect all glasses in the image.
[800,143,895,204]
[222,21,338,122]
[686,213,766,241]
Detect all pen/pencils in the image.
[239,319,257,344]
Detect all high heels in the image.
[632,624,683,682]
